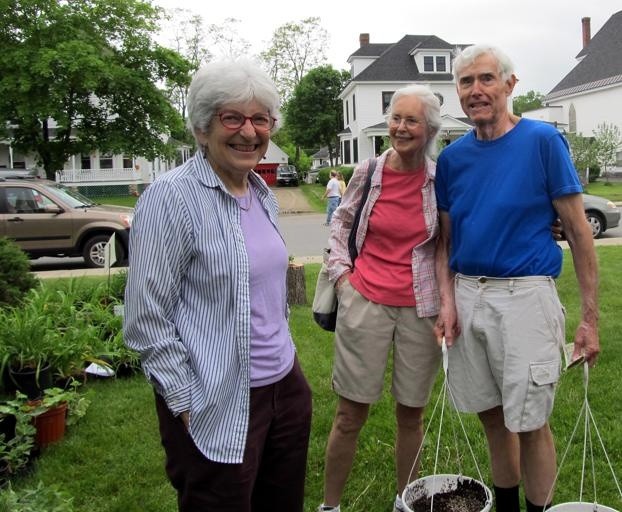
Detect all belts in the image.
[327,195,338,198]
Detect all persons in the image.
[431,41,600,511]
[123,59,314,512]
[321,170,347,226]
[314,82,562,512]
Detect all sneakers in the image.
[323,223,329,227]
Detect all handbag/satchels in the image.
[312,158,379,334]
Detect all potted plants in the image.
[0,286,89,476]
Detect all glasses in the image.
[213,109,278,134]
[386,115,427,129]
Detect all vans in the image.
[0,168,44,204]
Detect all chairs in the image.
[15,193,33,213]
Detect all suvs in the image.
[276,165,299,187]
[0,177,136,268]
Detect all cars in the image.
[554,193,621,239]
